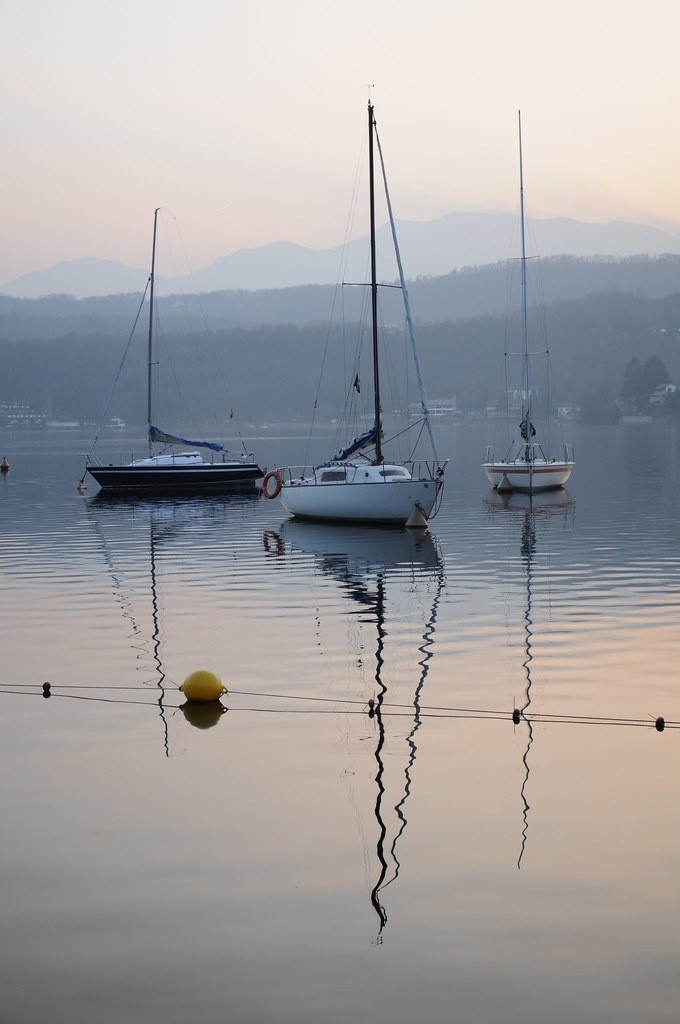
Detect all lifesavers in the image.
[263,471,281,499]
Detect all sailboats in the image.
[478,104,578,491]
[83,205,263,489]
[271,82,450,525]
